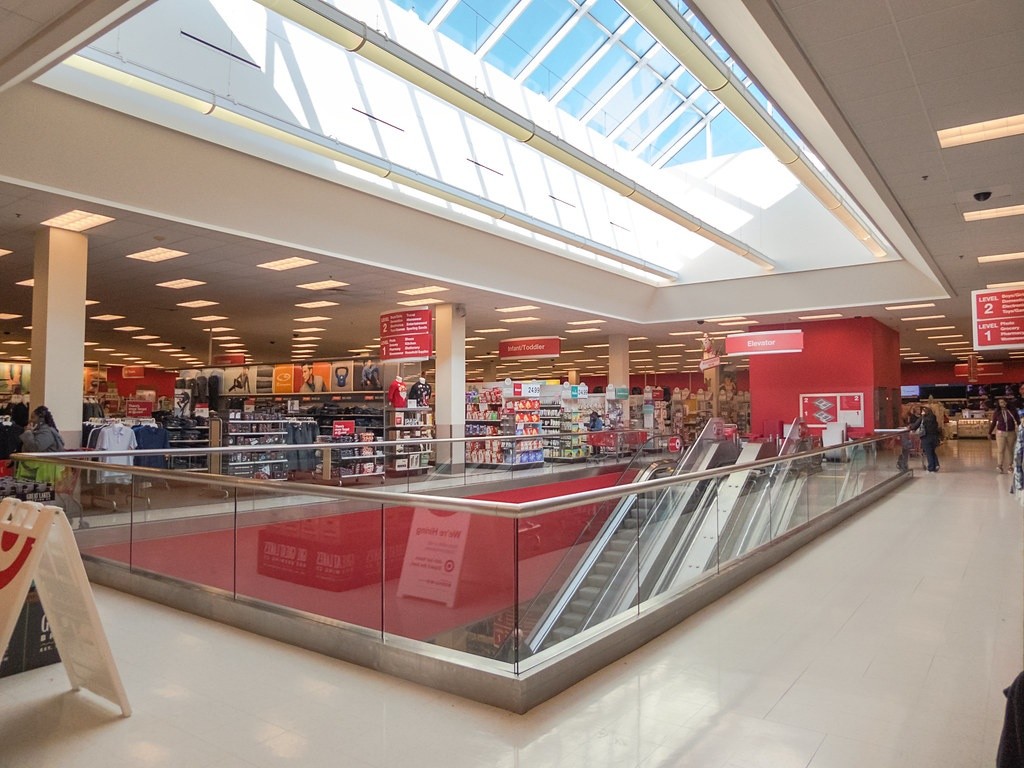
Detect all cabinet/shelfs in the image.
[383,407,434,478]
[501,388,545,471]
[465,402,506,469]
[218,390,389,429]
[224,419,289,481]
[311,442,386,486]
[166,426,209,472]
[559,389,589,464]
[540,404,562,463]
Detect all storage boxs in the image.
[393,445,404,453]
[407,455,419,468]
[421,414,433,425]
[389,412,404,425]
[0,592,61,678]
[560,412,590,458]
[388,431,396,441]
[465,391,544,463]
[395,430,411,439]
[392,458,408,469]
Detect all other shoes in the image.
[929,465,940,472]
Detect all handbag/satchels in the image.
[14,427,62,482]
[912,415,926,437]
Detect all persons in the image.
[909,406,940,473]
[19,406,65,453]
[1013,382,1024,490]
[508,629,533,664]
[409,378,431,408]
[177,390,190,415]
[361,360,382,391]
[300,362,327,393]
[587,412,602,455]
[229,367,250,394]
[987,399,1019,473]
[388,376,407,409]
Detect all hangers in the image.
[82,417,158,429]
[289,417,317,426]
[10,394,99,408]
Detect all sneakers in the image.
[996,466,1004,473]
[1007,466,1014,474]
